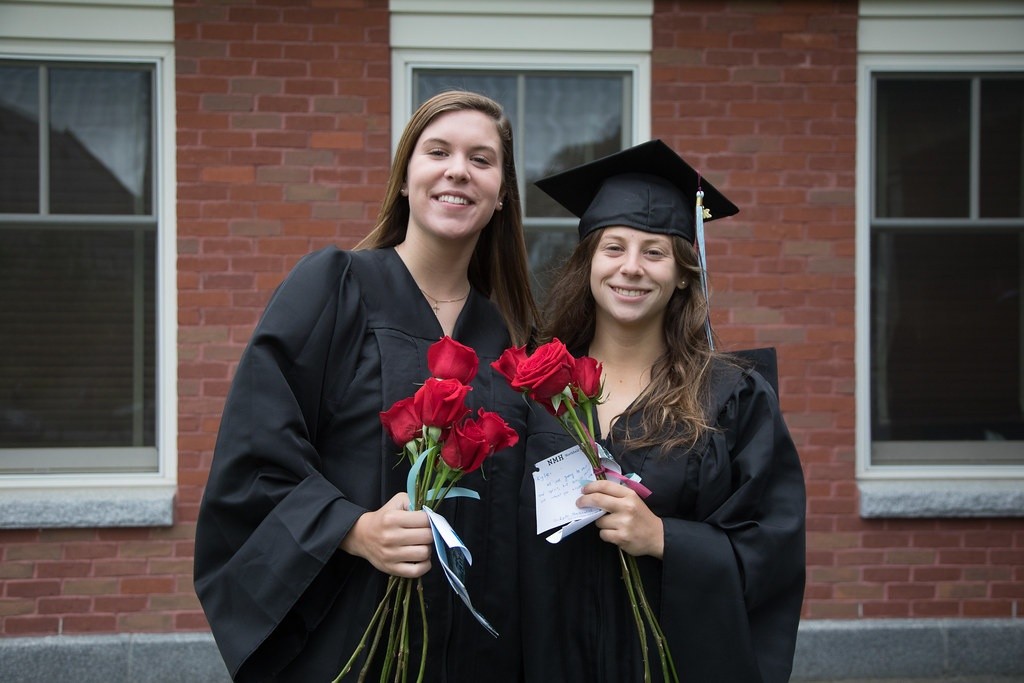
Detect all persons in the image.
[192,90,541,683]
[520,174,808,683]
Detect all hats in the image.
[534,138,740,354]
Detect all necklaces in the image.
[421,288,469,314]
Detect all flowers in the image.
[331,336,681,683]
